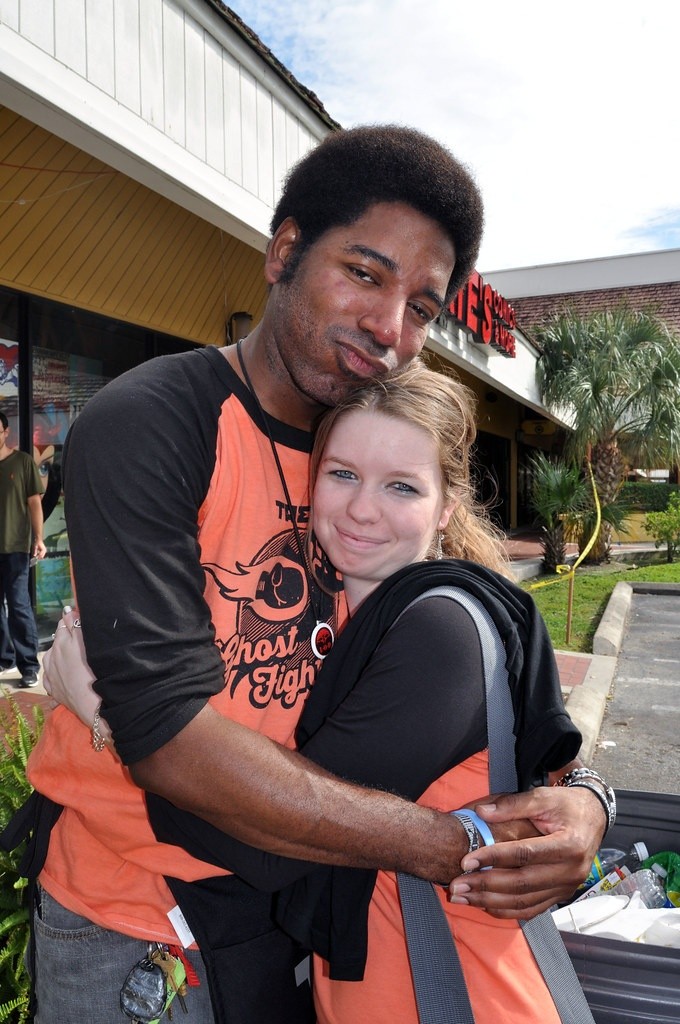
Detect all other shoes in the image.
[0,664,18,675]
[19,671,38,688]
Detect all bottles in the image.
[650,861,667,882]
[577,842,649,889]
[569,865,632,903]
[587,869,667,910]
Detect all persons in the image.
[40,363,579,1024]
[31,125,606,1024]
[0,412,47,689]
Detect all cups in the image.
[664,891,680,908]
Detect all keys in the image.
[121,951,188,1024]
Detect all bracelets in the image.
[91,704,106,752]
[559,768,616,835]
[438,808,495,889]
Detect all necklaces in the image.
[236,340,338,656]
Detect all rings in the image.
[51,626,66,639]
[63,605,72,615]
[71,619,82,632]
